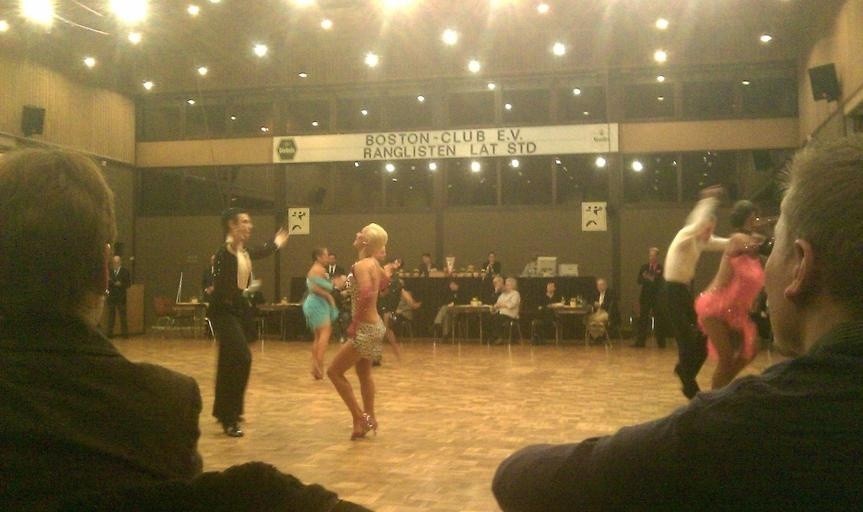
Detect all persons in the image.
[690,197,768,391]
[325,253,347,342]
[0,146,207,512]
[663,180,730,402]
[375,245,421,361]
[482,251,501,293]
[529,280,561,345]
[581,278,615,342]
[210,206,290,438]
[432,280,471,344]
[413,252,437,277]
[102,250,134,344]
[325,223,389,440]
[481,276,521,345]
[630,247,665,348]
[303,248,340,381]
[200,253,218,337]
[85,129,860,510]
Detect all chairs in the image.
[151,296,624,348]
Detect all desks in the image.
[291,276,598,337]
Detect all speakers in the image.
[809,63,839,102]
[21,105,45,137]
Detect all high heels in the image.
[350,411,378,439]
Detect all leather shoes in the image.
[220,420,243,438]
